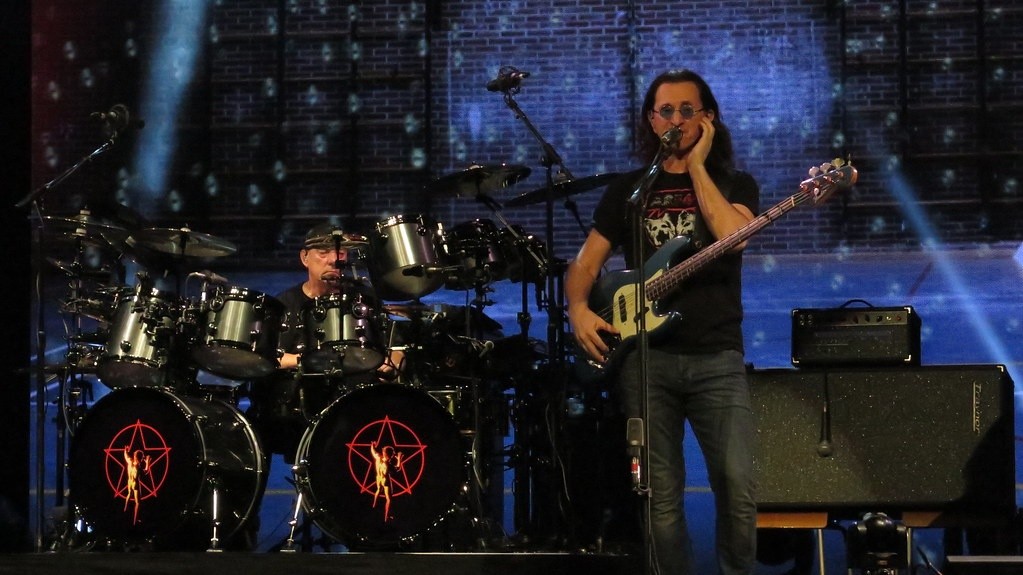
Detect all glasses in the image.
[649,104,707,120]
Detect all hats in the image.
[304,224,346,249]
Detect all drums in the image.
[298,293,387,374]
[294,382,471,549]
[98,289,197,396]
[194,385,239,407]
[187,285,285,382]
[445,219,545,291]
[66,383,268,550]
[428,381,513,439]
[365,213,445,302]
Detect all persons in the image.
[564,67,759,575]
[275,220,406,383]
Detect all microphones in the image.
[818,403,833,456]
[487,72,531,93]
[333,226,342,252]
[662,128,683,145]
[100,112,145,130]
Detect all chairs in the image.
[754,509,830,575]
[902,508,956,573]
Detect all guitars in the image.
[577,158,858,389]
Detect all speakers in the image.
[746,365,1016,516]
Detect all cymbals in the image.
[27,214,130,246]
[133,228,237,259]
[439,164,532,199]
[305,232,371,249]
[18,364,97,379]
[503,172,624,209]
